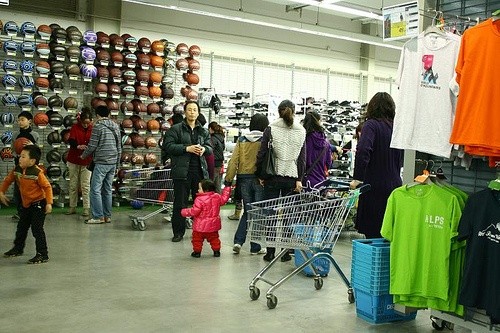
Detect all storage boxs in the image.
[294,222,419,325]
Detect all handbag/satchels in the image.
[259,126,275,179]
[86,160,95,171]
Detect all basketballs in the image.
[14,137,30,155]
[19,75,35,88]
[1,92,18,106]
[33,95,48,108]
[0,112,15,126]
[38,163,47,177]
[35,77,49,91]
[20,40,35,54]
[20,59,34,72]
[36,23,201,210]
[4,40,19,53]
[4,20,19,35]
[34,113,49,127]
[0,131,15,145]
[2,59,18,72]
[0,20,3,34]
[21,22,37,36]
[0,147,15,162]
[2,74,18,87]
[18,93,33,108]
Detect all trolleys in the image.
[247,178,371,310]
[121,165,193,231]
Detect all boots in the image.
[229,209,241,220]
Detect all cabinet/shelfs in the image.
[0,34,369,206]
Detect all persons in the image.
[163,101,214,242]
[209,121,225,194]
[257,100,306,261]
[66,106,94,215]
[224,114,270,255]
[349,92,404,239]
[331,145,342,154]
[304,111,334,223]
[0,145,53,263]
[228,178,242,220]
[181,179,231,257]
[81,105,121,223]
[12,111,40,218]
[159,113,193,228]
[197,113,215,180]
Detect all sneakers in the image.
[27,254,49,263]
[252,248,266,255]
[4,249,23,256]
[233,244,241,253]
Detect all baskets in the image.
[292,224,332,277]
[352,239,418,325]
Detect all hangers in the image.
[393,6,500,201]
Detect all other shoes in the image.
[263,254,274,262]
[214,91,268,169]
[281,255,292,262]
[172,235,183,242]
[289,252,295,256]
[83,212,89,216]
[295,97,369,161]
[164,216,172,221]
[191,251,200,257]
[84,217,105,224]
[12,214,21,223]
[105,217,111,222]
[65,211,76,215]
[214,251,220,257]
